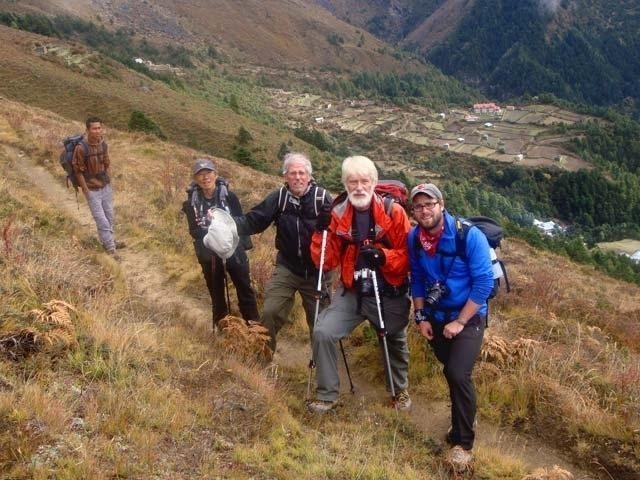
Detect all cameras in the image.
[424,280,449,308]
[353,268,374,297]
[94,171,110,183]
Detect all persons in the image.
[59,117,117,253]
[207,153,334,370]
[310,156,413,412]
[408,184,504,469]
[182,160,261,333]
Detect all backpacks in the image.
[329,179,412,213]
[60,135,85,189]
[453,214,503,299]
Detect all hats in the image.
[192,161,215,176]
[411,184,443,201]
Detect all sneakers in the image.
[308,399,338,415]
[447,419,479,435]
[115,241,126,248]
[449,445,474,474]
[108,249,120,260]
[395,390,411,410]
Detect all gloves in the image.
[316,204,332,232]
[362,249,385,270]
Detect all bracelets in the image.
[455,320,467,326]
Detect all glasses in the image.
[411,200,439,212]
